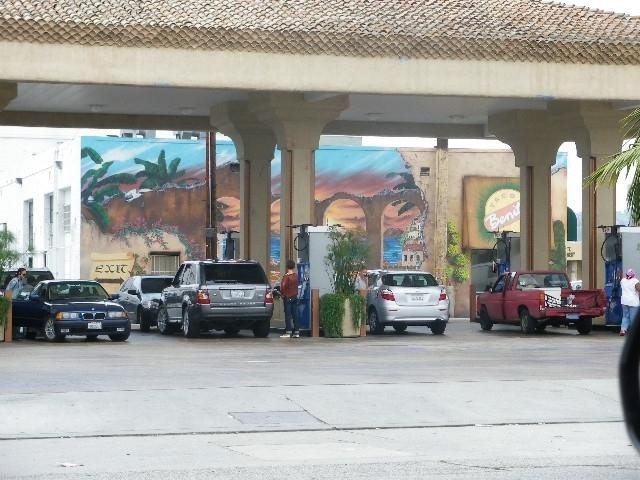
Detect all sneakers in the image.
[619,331,625,336]
[280,334,291,338]
[292,335,300,338]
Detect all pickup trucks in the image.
[472,268,607,336]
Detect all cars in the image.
[0,266,54,292]
[2,279,134,344]
[367,268,449,335]
[118,274,174,330]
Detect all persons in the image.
[4,268,27,300]
[277,261,302,338]
[617,268,640,337]
[355,266,371,291]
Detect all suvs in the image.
[157,257,275,336]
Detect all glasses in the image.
[21,274,26,276]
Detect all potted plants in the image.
[320,220,368,338]
